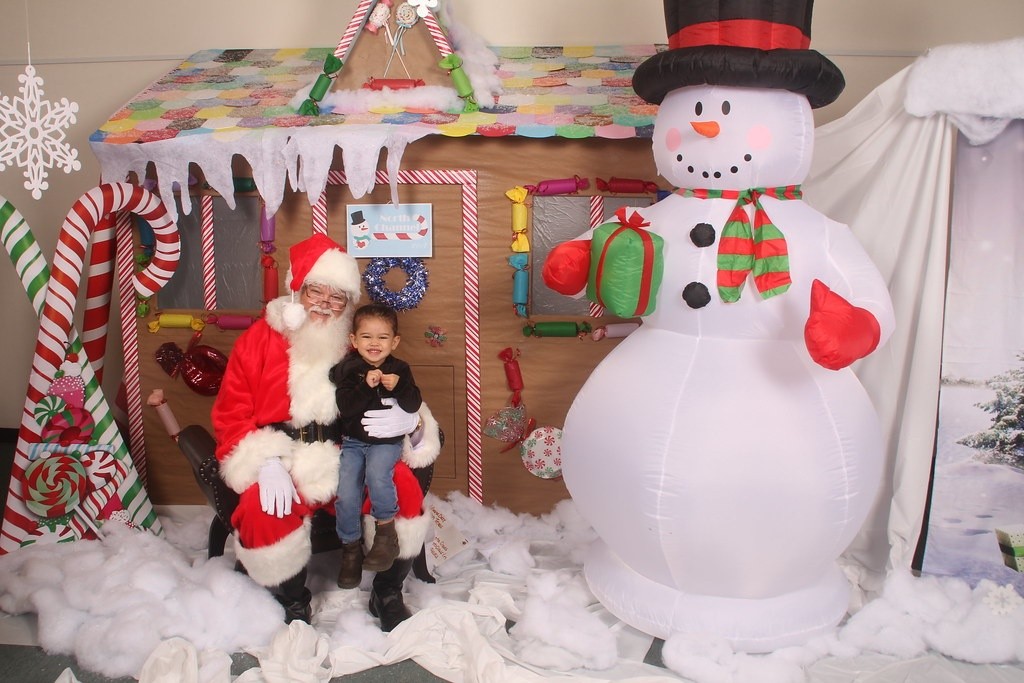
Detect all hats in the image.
[288,231,360,290]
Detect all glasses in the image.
[303,286,349,311]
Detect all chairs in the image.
[177,423,445,584]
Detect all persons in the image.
[329,305,423,589]
[212,261,439,634]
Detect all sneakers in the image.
[364,534,399,572]
[337,547,363,589]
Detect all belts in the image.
[270,421,343,449]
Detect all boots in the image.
[234,517,314,626]
[367,505,428,633]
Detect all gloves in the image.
[257,456,301,519]
[361,397,420,439]
